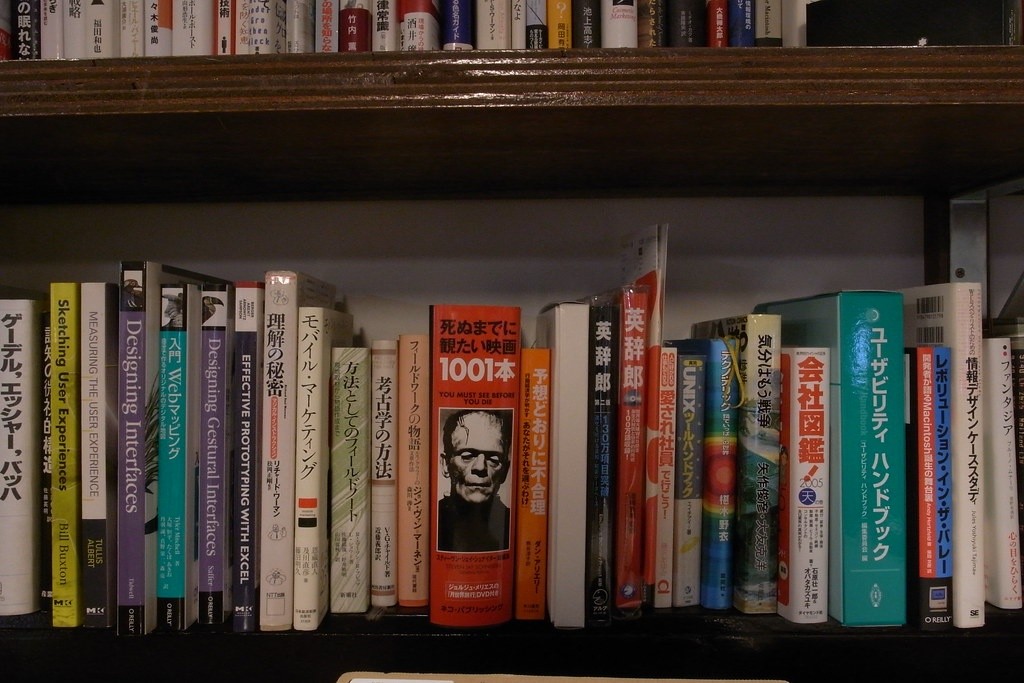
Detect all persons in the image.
[438,407,511,555]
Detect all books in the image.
[0,248,1024,630]
[0,0,811,68]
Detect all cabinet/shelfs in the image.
[0,50,1024,683]
